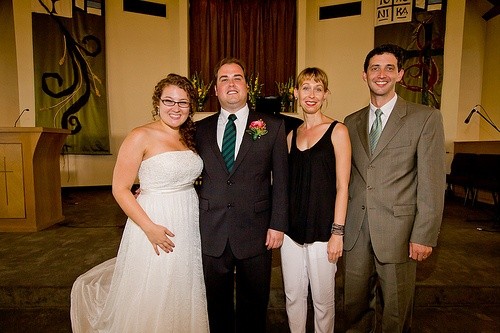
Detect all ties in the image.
[368,108,384,157]
[222,114,237,174]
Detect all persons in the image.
[342,45,446,333]
[134,57,287,333]
[71,72,213,333]
[279,66,352,333]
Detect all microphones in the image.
[464,105,477,123]
[15,109,29,128]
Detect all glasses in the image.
[158,96,192,108]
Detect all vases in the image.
[280,96,287,112]
[197,98,204,113]
[249,94,257,111]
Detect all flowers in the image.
[248,118,268,140]
[275,75,296,108]
[191,70,215,110]
[247,72,265,108]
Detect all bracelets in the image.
[331,222,345,237]
